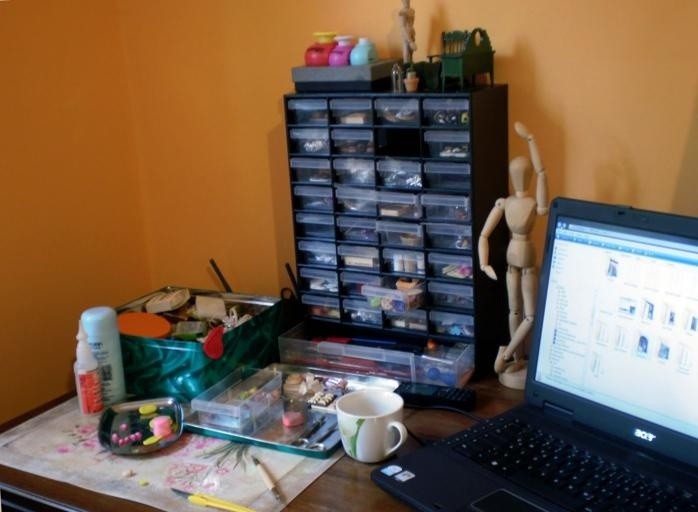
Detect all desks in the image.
[1,371,527,512]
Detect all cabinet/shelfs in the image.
[275,83,512,390]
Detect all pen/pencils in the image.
[174,488,254,512]
[251,455,283,505]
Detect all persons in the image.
[398,0,417,64]
[477,121,548,390]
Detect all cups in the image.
[334,389,408,462]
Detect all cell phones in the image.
[394,381,477,412]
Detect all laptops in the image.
[369,196,698,512]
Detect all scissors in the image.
[276,415,338,452]
